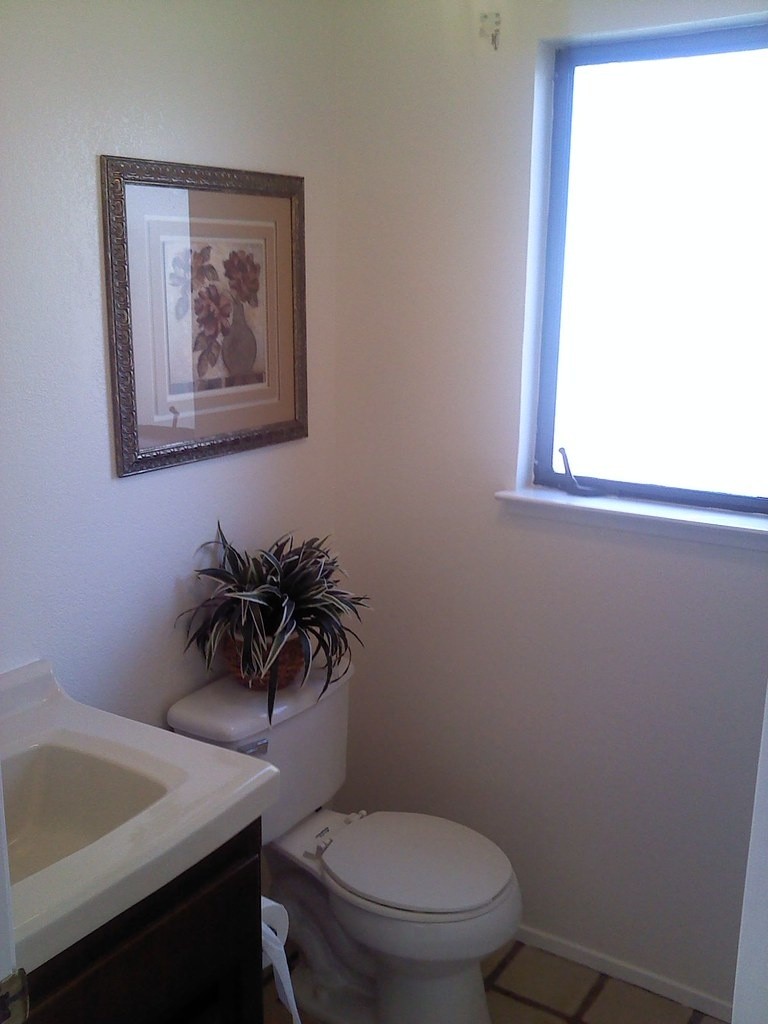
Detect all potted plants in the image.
[170,518,372,723]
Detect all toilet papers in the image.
[262,895,303,1023]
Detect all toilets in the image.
[165,649,523,1024]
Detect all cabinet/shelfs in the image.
[24,819,264,1024]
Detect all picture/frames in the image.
[97,154,309,479]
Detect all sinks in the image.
[1,742,171,887]
